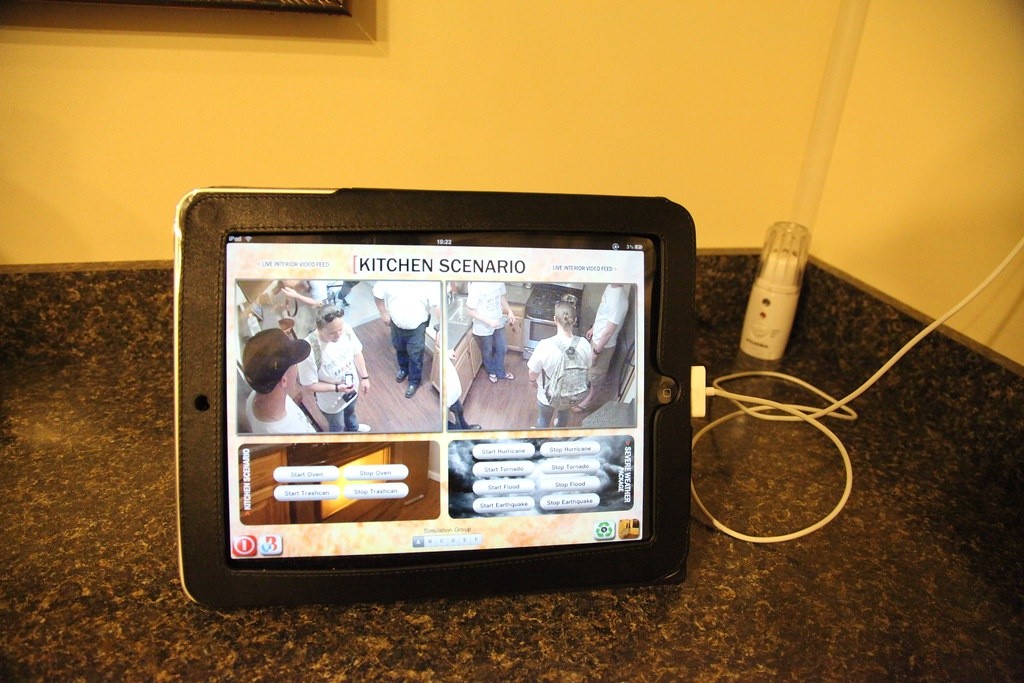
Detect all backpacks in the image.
[542,333,591,410]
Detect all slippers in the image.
[489,373,497,383]
[503,373,514,379]
[572,402,591,413]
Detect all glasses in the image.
[317,309,344,324]
[554,300,576,315]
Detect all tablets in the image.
[173,184,698,615]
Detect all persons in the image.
[464,281,517,385]
[268,279,327,339]
[243,328,317,433]
[372,280,442,398]
[528,295,592,430]
[571,284,629,412]
[297,303,370,432]
[447,349,481,429]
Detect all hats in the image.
[243,328,311,395]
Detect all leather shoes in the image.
[396,369,408,382]
[405,383,419,398]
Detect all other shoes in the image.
[356,423,371,432]
